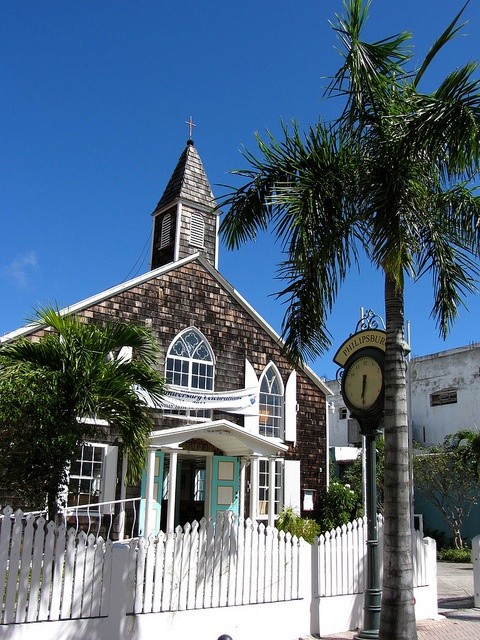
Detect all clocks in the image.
[342,349,384,417]
